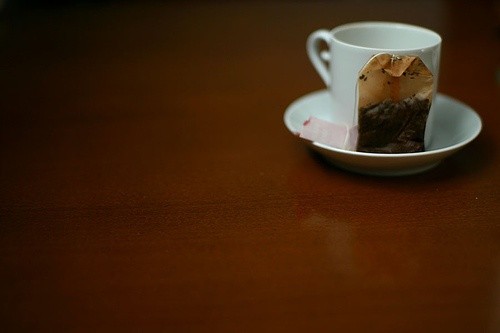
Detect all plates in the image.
[284,88,482,176]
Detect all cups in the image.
[306,22,442,148]
